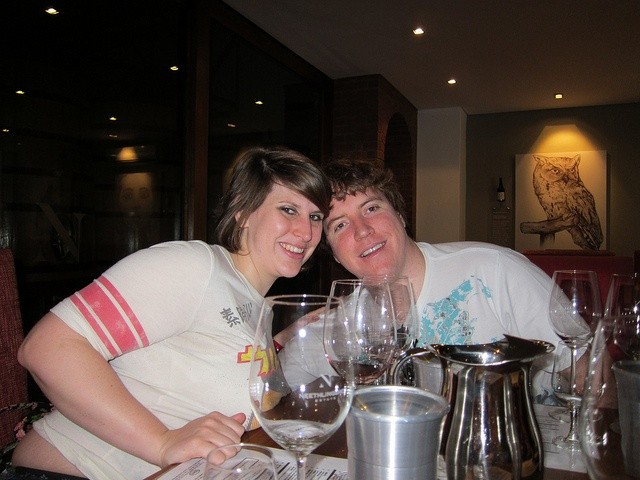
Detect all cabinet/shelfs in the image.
[497,176,505,202]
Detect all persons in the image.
[277,155,616,410]
[7,147,330,480]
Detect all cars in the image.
[577,315,640,480]
[201,444,278,480]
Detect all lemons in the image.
[388,333,555,479]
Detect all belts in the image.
[355,274,416,386]
[323,277,399,385]
[548,269,604,423]
[551,339,591,453]
[247,293,355,479]
[605,273,640,436]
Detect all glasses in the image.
[495,177,506,203]
